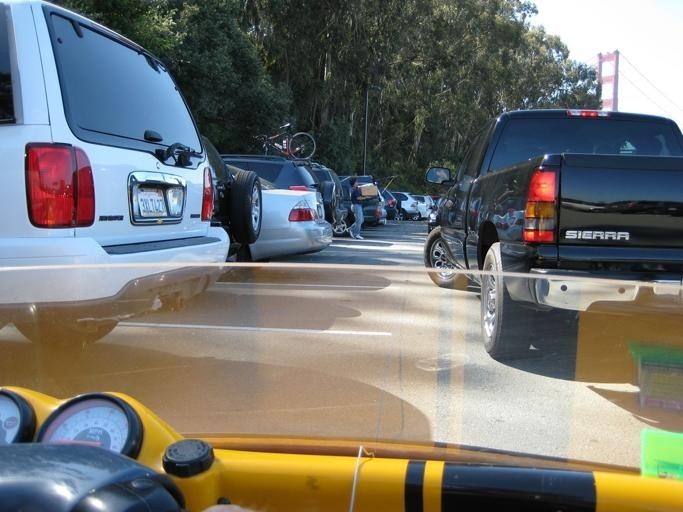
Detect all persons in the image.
[345,178,371,239]
[448,196,524,229]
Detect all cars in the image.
[389,192,418,222]
[377,188,397,222]
[375,187,387,226]
[217,153,320,193]
[222,164,333,283]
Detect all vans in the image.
[0,2,229,348]
[331,175,382,237]
[308,162,348,226]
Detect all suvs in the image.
[408,194,437,221]
[199,135,262,259]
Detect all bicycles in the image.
[252,122,316,161]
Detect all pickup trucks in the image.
[423,108,682,360]
